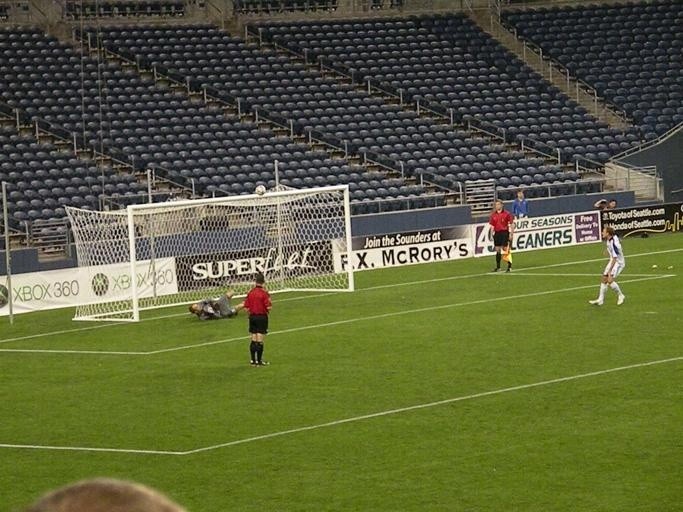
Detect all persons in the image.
[512,189,530,219]
[243,273,272,368]
[23,478,182,512]
[588,227,625,306]
[593,199,617,210]
[189,289,246,321]
[488,199,515,274]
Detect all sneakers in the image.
[492,268,501,272]
[251,360,269,367]
[617,295,625,305]
[588,299,603,305]
[506,268,511,273]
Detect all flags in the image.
[502,244,513,263]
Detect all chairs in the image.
[0,0,682,256]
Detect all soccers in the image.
[255,185,265,194]
